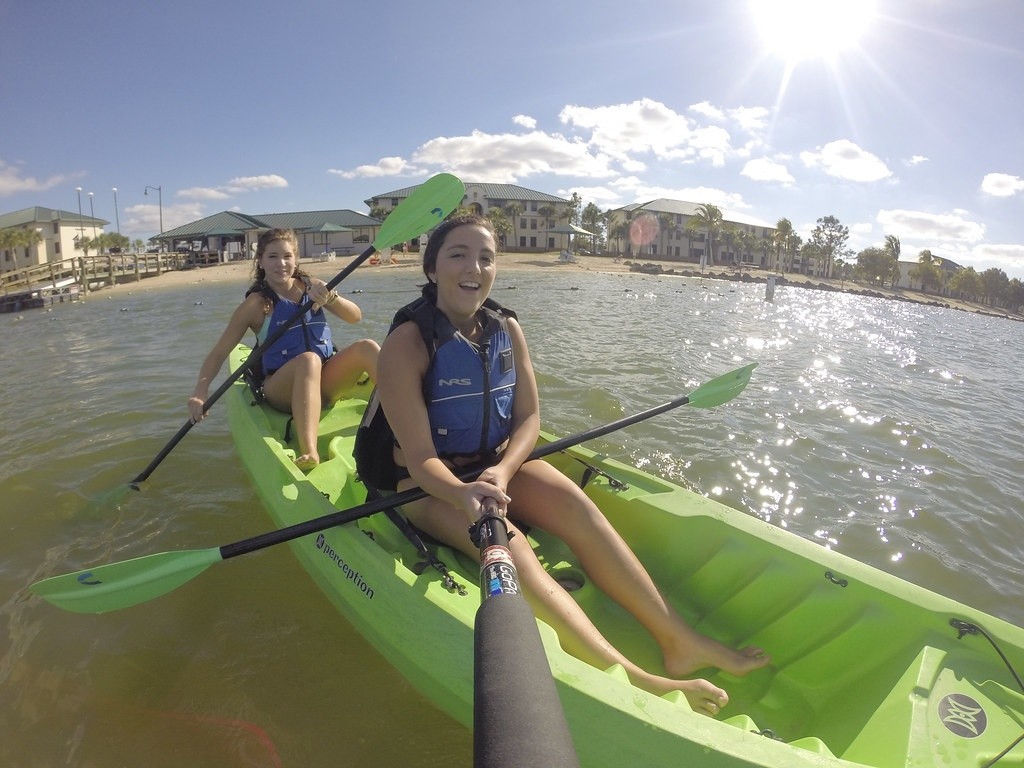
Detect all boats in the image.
[225,343,1024,768]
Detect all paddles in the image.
[27,362,760,615]
[130,172,466,480]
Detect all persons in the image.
[376,210,767,715]
[402,242,408,257]
[187,229,381,472]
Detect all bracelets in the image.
[328,292,338,303]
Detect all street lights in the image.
[75,187,84,239]
[144,186,164,253]
[87,192,99,255]
[112,187,122,253]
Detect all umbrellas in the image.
[540,224,597,252]
